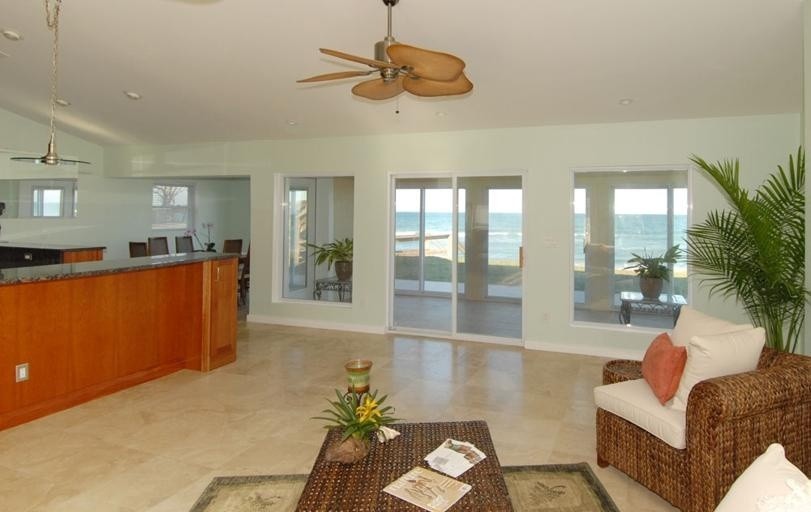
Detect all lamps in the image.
[9,0,91,167]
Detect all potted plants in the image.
[622,239,685,298]
[308,236,353,280]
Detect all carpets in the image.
[191,461,620,512]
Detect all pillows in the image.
[670,327,766,410]
[713,443,811,512]
[640,332,687,407]
[671,304,753,347]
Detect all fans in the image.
[295,0,474,101]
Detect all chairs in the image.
[237,245,250,306]
[595,347,811,512]
[128,236,195,256]
[223,239,242,255]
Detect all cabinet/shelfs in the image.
[184,258,238,372]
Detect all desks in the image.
[619,291,687,327]
[312,276,352,302]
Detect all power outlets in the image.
[15,362,29,383]
